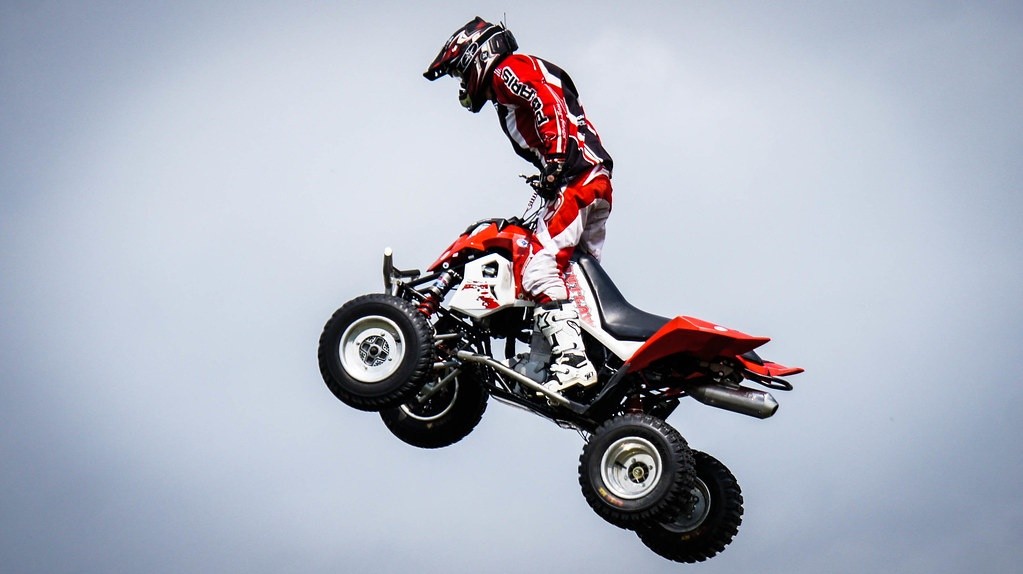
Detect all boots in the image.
[534,299,597,398]
[495,333,551,399]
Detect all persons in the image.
[421,16,613,400]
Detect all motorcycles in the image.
[318,162,804,562]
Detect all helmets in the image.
[423,16,520,113]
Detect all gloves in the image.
[540,165,563,198]
